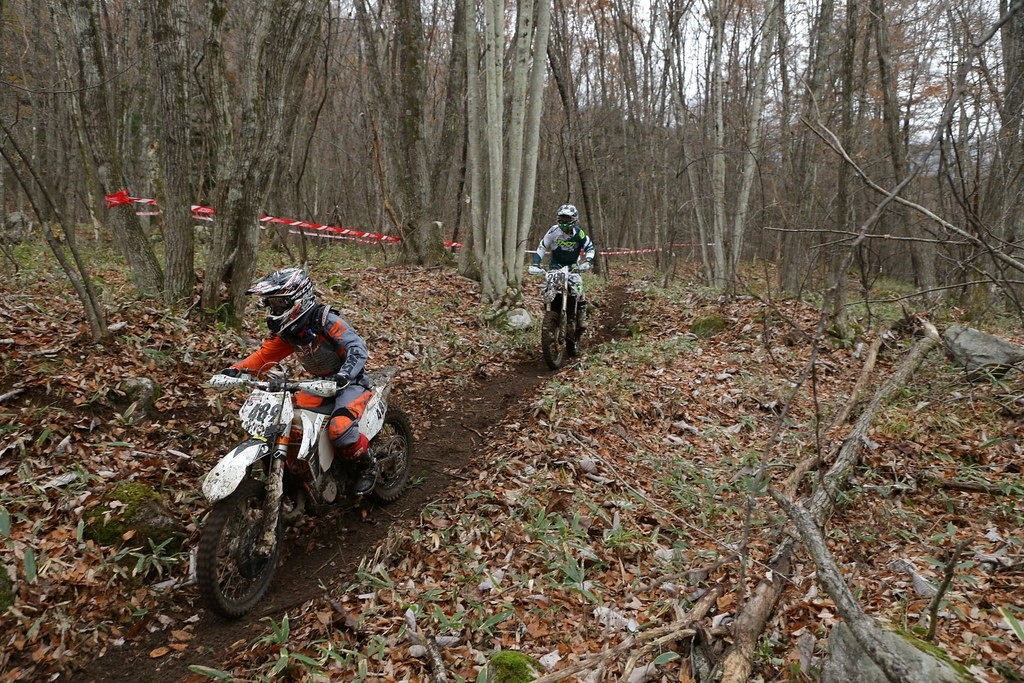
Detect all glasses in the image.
[558,220,570,224]
[263,296,295,311]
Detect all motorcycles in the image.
[528,262,590,371]
[197,362,416,623]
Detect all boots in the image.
[354,447,377,496]
[577,300,589,328]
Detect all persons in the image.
[532,203,595,328]
[221,267,379,494]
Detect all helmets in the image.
[556,204,578,231]
[245,268,316,337]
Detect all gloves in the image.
[325,373,348,388]
[528,264,541,276]
[221,367,241,378]
